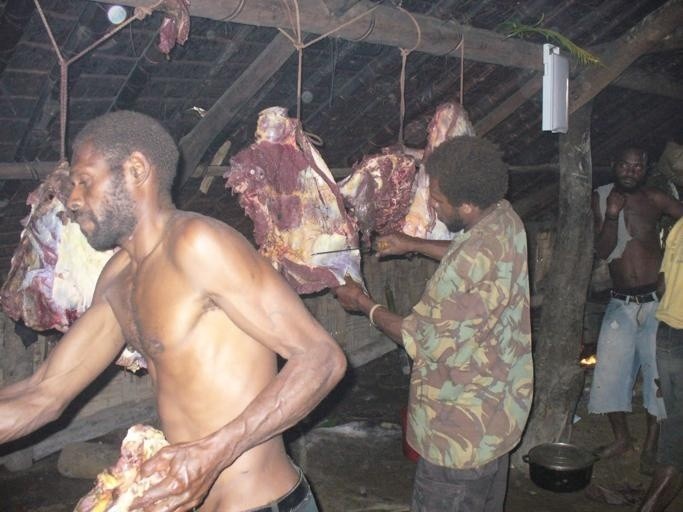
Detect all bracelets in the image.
[369,304,387,327]
[605,213,618,221]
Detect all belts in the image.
[254,473,309,512]
[610,292,660,302]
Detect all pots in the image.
[522,442,601,494]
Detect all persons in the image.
[586,142,683,477]
[638,215,683,512]
[0,109,347,512]
[331,134,534,512]
